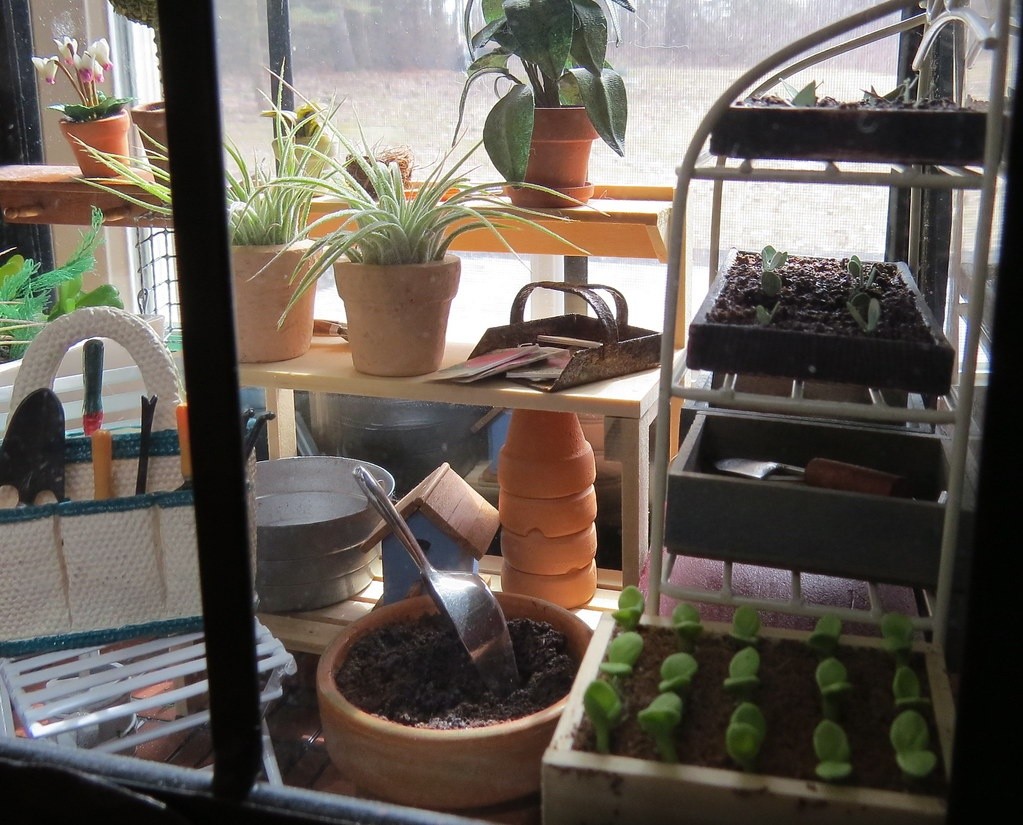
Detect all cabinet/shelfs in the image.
[231,335,662,662]
[634,0,1010,646]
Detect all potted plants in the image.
[235,65,613,377]
[65,55,363,366]
[452,0,638,188]
[683,242,956,399]
[701,70,982,161]
[258,101,332,190]
[534,580,959,824]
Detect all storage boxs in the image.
[660,406,978,592]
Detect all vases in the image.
[58,108,128,180]
[129,101,171,187]
[312,587,598,824]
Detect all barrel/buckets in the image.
[249,451,394,614]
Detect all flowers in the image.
[28,35,138,122]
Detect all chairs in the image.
[2,344,290,785]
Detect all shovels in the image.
[352,464,523,698]
[0,388,68,510]
